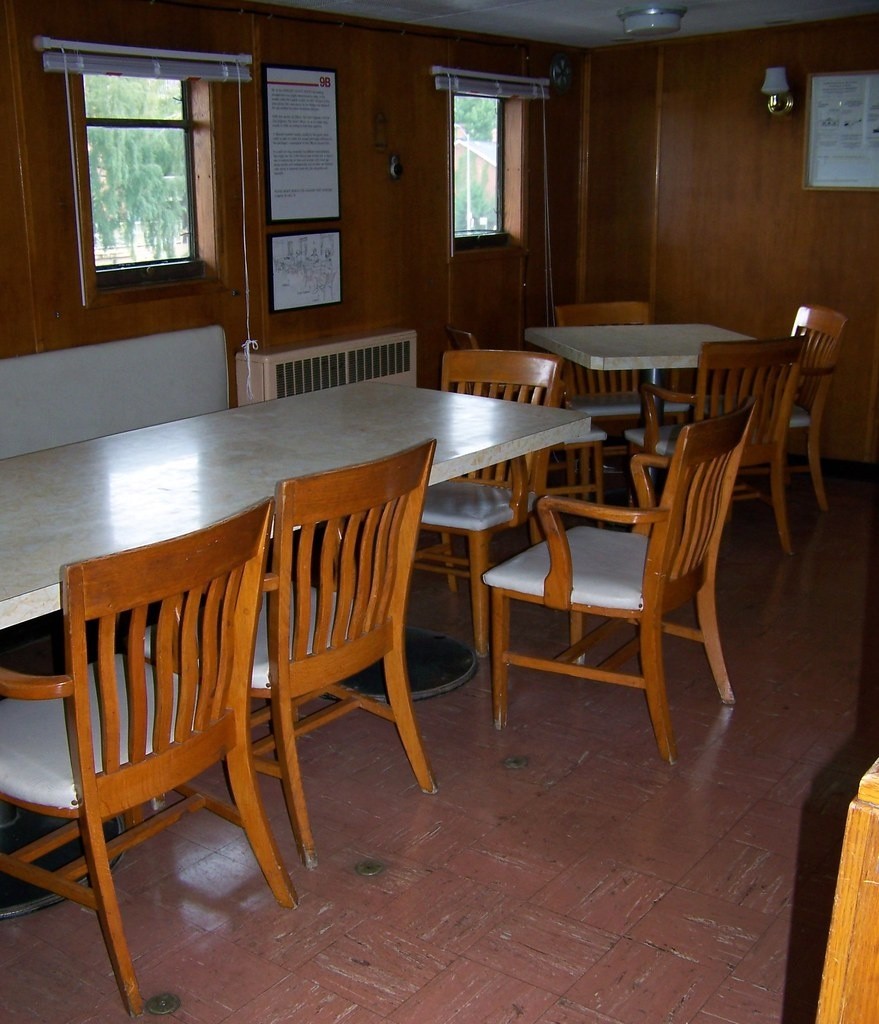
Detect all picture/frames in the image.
[265,228,343,315]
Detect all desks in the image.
[523,324,759,536]
[0,379,591,919]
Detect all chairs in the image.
[449,325,608,532]
[557,301,690,502]
[411,351,565,662]
[128,438,439,871]
[623,334,811,561]
[744,305,848,514]
[0,498,300,1017]
[483,396,757,768]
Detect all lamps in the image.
[616,4,688,35]
[760,67,800,117]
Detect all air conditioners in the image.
[238,327,417,407]
